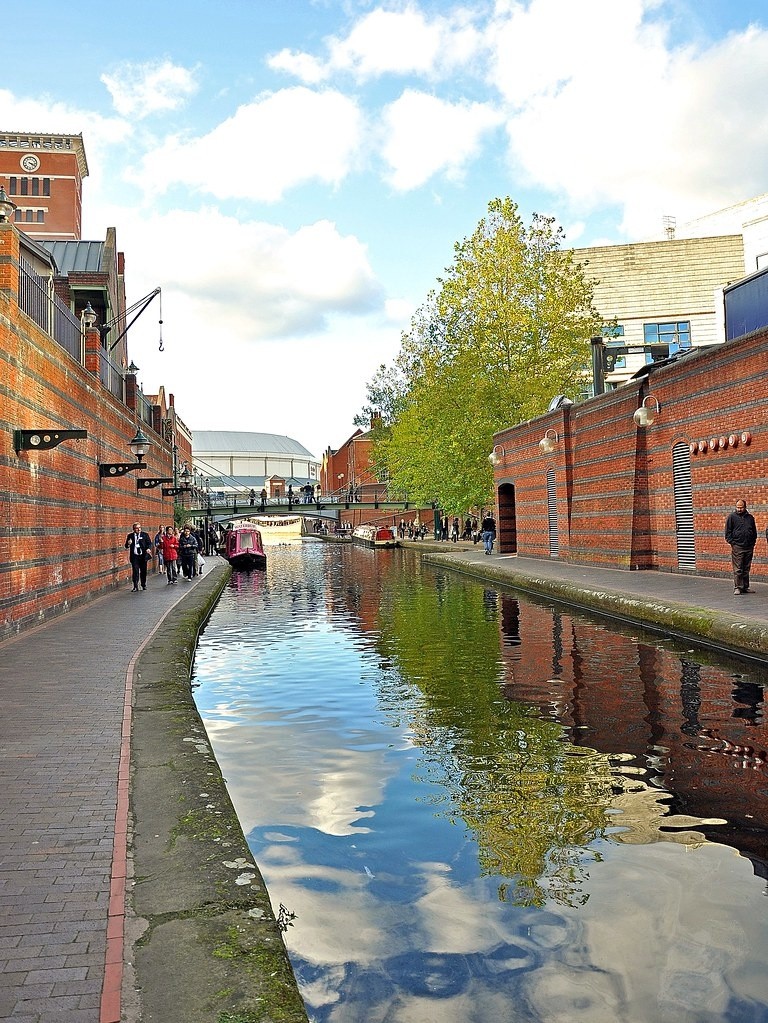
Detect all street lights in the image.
[337,475,341,488]
[339,472,345,493]
[184,460,210,493]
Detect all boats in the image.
[350,522,395,549]
[219,523,266,564]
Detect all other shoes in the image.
[734,588,740,595]
[131,587,138,592]
[174,580,178,583]
[169,581,173,584]
[143,587,147,590]
[188,579,192,582]
[743,588,756,593]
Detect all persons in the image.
[249,488,255,506]
[304,481,312,503]
[125,522,153,592]
[155,523,206,585]
[311,485,317,503]
[354,483,360,503]
[398,515,484,544]
[314,520,354,539]
[481,511,496,556]
[260,489,267,506]
[725,499,757,595]
[349,482,353,502]
[288,484,294,505]
[209,526,219,556]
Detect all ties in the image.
[136,535,141,555]
